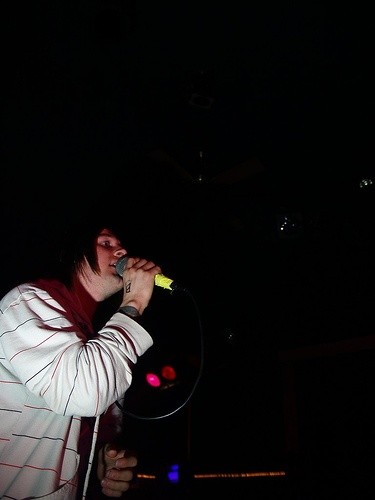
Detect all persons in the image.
[1,215,162,500]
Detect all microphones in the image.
[115,256,178,290]
